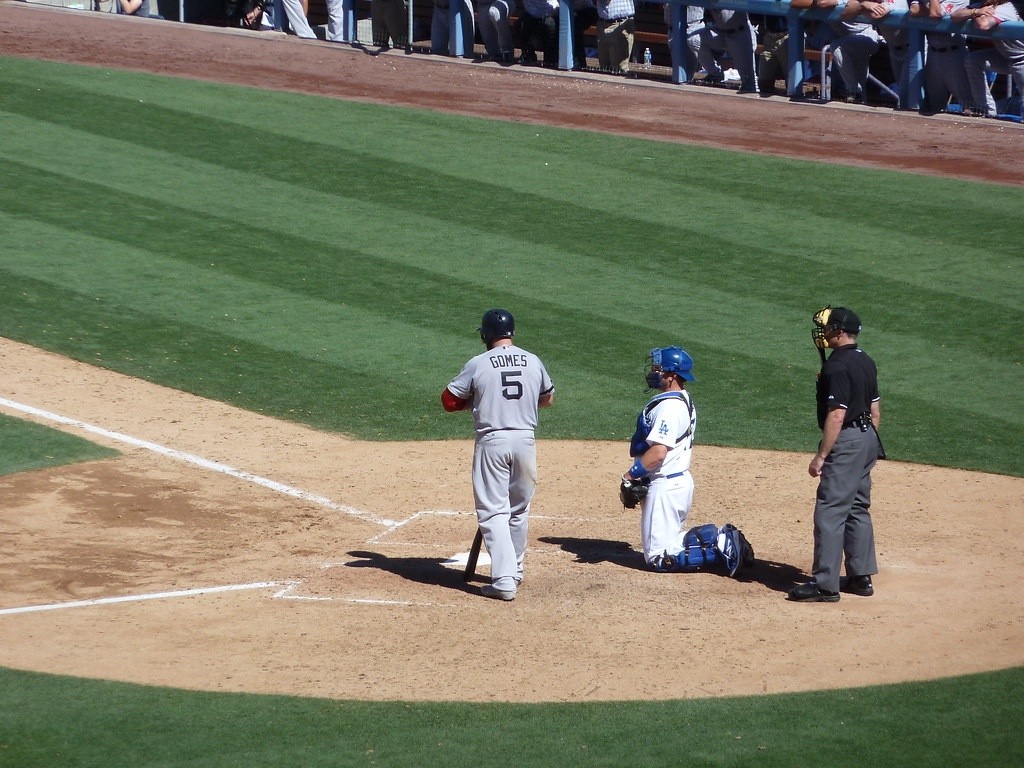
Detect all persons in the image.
[96,0,1024,121]
[619,346,754,580]
[442,309,555,602]
[789,306,880,603]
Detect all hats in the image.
[830,307,862,333]
[656,347,696,381]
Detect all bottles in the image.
[643,48,652,69]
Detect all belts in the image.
[896,44,911,51]
[666,472,683,479]
[727,26,744,34]
[599,16,630,23]
[930,46,958,54]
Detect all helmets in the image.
[479,307,518,343]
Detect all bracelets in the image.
[972,8,977,17]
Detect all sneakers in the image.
[848,576,874,596]
[721,523,756,578]
[481,585,516,600]
[788,585,841,603]
